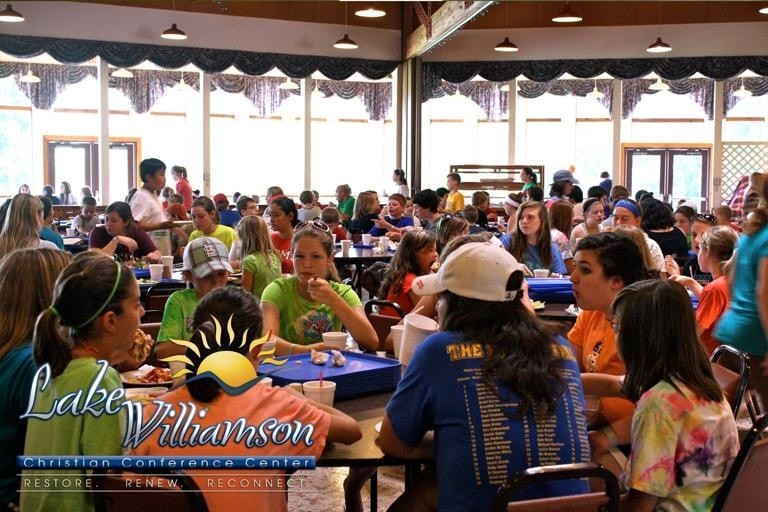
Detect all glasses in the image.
[690,214,717,224]
[293,221,331,236]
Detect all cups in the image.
[399,313,439,368]
[498,216,504,227]
[162,256,175,279]
[65,228,75,236]
[391,325,405,360]
[340,240,352,256]
[304,380,336,407]
[533,268,550,278]
[331,234,336,245]
[361,233,371,246]
[322,331,346,350]
[380,236,389,251]
[259,377,273,388]
[147,387,167,398]
[141,256,150,270]
[149,263,163,282]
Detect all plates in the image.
[124,387,153,400]
[117,369,174,386]
[534,304,545,310]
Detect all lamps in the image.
[0,1,768,55]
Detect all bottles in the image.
[79,233,84,245]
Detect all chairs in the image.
[1,200,767,511]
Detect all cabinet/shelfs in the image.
[449,164,543,209]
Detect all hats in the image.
[553,170,579,184]
[182,236,234,279]
[214,194,227,206]
[410,242,526,302]
